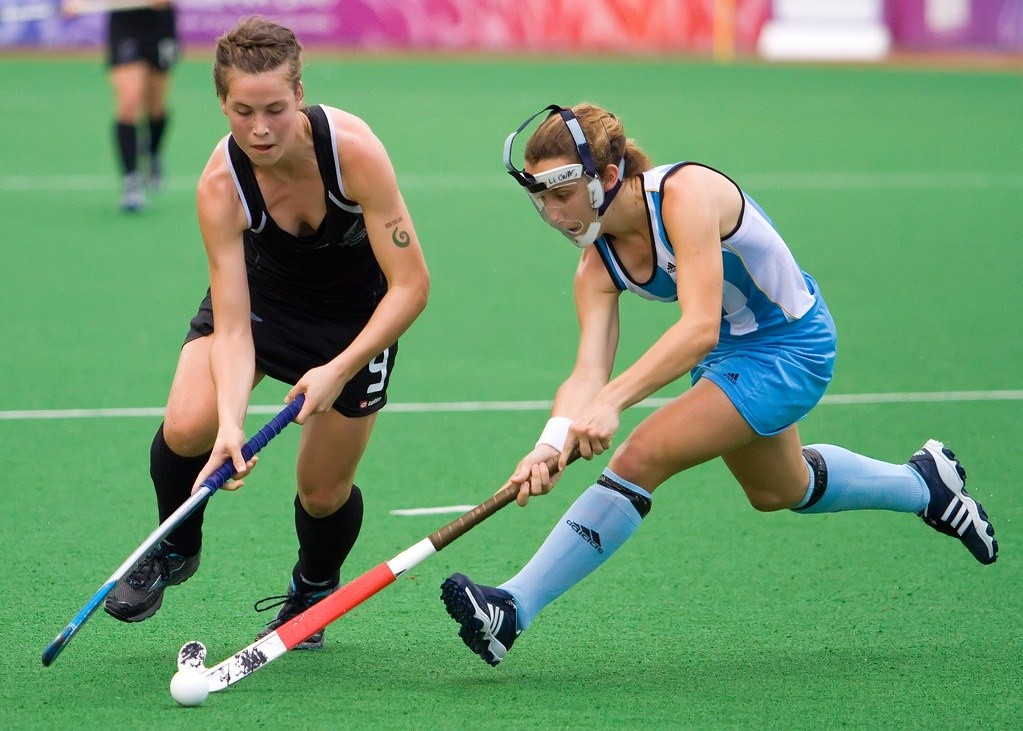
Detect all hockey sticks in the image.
[41,390,306,666]
[177,438,596,694]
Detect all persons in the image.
[105,4,185,215]
[438,98,1001,666]
[99,11,434,651]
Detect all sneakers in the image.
[440,572,522,667]
[104,540,201,623]
[255,560,339,649]
[908,438,998,565]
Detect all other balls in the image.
[170,669,212,709]
